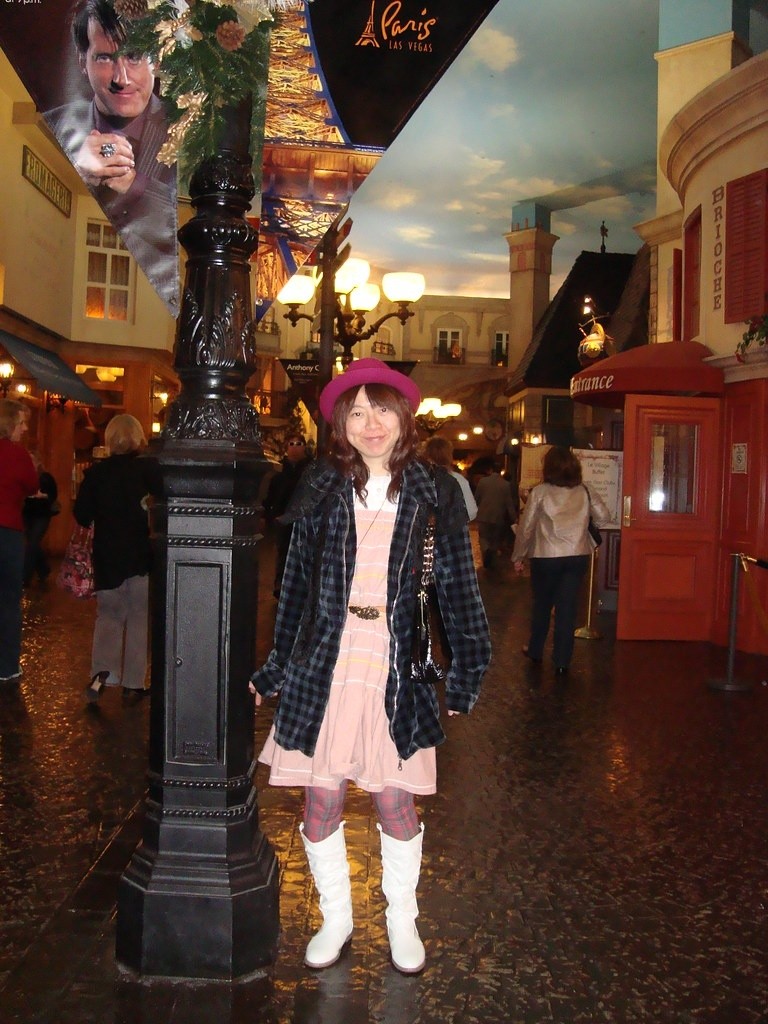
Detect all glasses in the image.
[288,441,304,446]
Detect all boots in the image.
[376,821,427,973]
[299,820,354,968]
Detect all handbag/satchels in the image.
[412,470,453,682]
[589,520,602,545]
[54,523,95,600]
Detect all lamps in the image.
[0,353,15,397]
[583,296,599,318]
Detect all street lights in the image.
[276,254,427,369]
[417,396,462,436]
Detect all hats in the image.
[319,358,422,423]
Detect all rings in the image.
[99,144,117,158]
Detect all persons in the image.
[259,432,314,599]
[23,451,57,585]
[73,415,162,698]
[511,446,612,676]
[41,0,181,320]
[424,432,522,569]
[0,398,39,681]
[249,357,491,973]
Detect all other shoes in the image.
[0,664,23,680]
[121,689,144,706]
[87,671,110,700]
[483,548,493,568]
[552,662,567,674]
[522,645,542,664]
